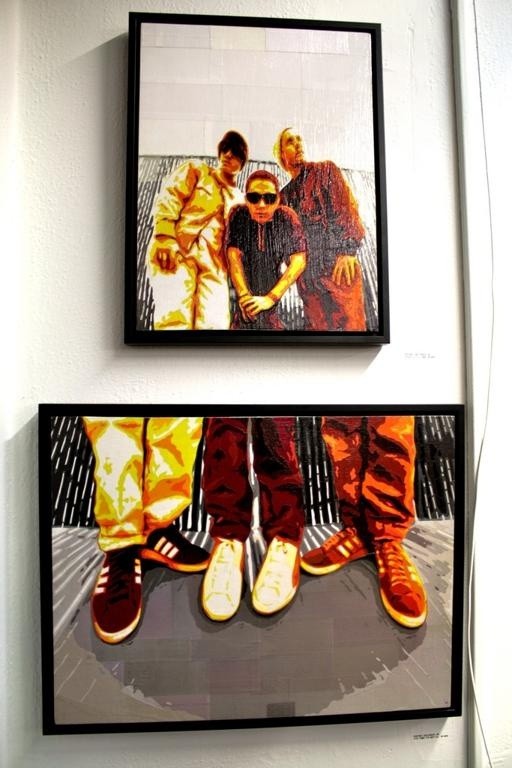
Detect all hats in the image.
[272,126,297,174]
[217,131,249,171]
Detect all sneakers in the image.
[300,525,377,576]
[251,535,301,614]
[92,542,144,647]
[202,537,247,623]
[373,538,428,629]
[140,522,210,572]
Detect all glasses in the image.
[246,192,278,204]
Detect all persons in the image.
[196,418,306,622]
[76,417,216,643]
[272,125,369,329]
[298,415,426,633]
[228,172,310,329]
[146,130,249,332]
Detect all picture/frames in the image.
[121,9,389,350]
[31,397,463,732]
